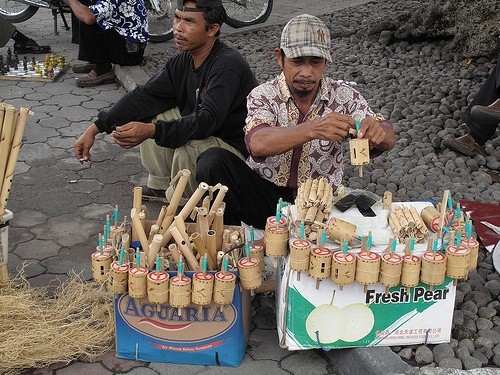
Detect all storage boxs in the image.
[114,220,266,367]
[276,201,458,351]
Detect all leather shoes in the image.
[443,134,493,156]
[470,99,500,126]
[12,39,51,54]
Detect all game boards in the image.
[0,60,70,82]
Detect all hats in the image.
[175,1,225,12]
[280,14,332,62]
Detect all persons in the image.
[75,0,260,223]
[0,15,52,54]
[197,14,396,230]
[61,0,149,87]
[442,37,500,159]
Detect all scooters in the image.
[222,0,273,28]
[0,0,183,43]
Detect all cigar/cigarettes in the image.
[77,157,85,162]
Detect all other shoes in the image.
[133,184,171,203]
[72,60,114,73]
[75,70,115,87]
